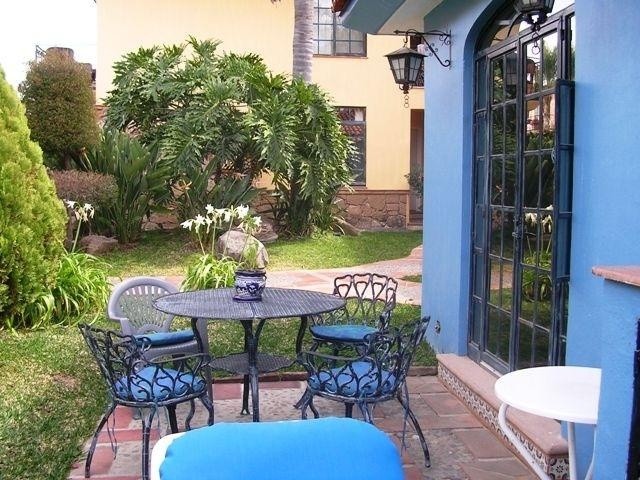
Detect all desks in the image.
[493,365,603,480]
[152,288,347,423]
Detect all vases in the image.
[233,269,267,301]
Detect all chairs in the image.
[149,417,406,480]
[106,275,214,404]
[294,315,433,468]
[296,273,399,378]
[77,323,215,480]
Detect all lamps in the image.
[384,29,452,110]
[510,1,558,56]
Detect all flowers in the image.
[179,196,262,288]
[515,201,556,300]
[52,198,113,294]
